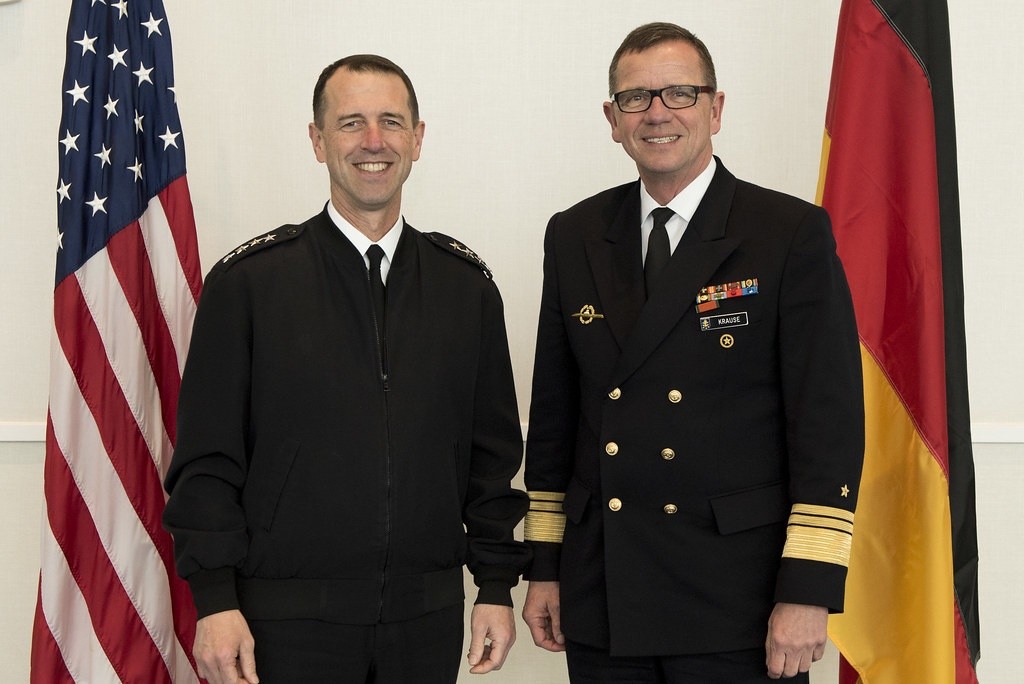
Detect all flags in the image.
[815,0,983,684]
[28,0,204,684]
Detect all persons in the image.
[163,54,529,684]
[523,18,865,684]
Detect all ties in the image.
[643,208,675,299]
[367,243,386,378]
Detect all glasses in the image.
[610,84,716,114]
